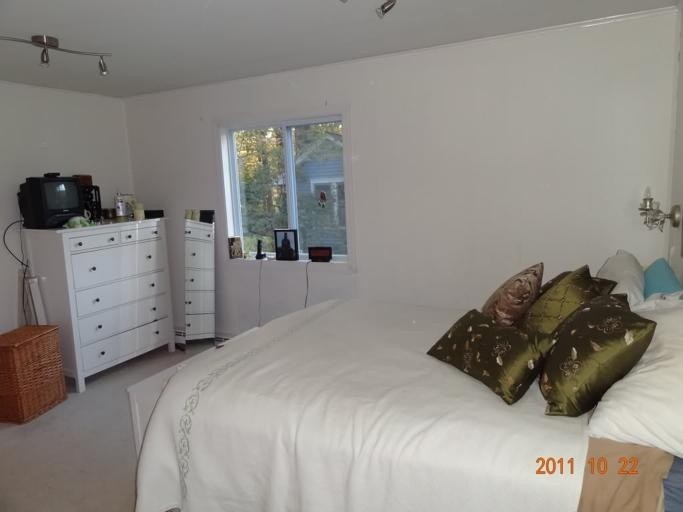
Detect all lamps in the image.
[99,55,107,75]
[41,48,50,66]
[376,0,395,15]
[638,193,681,232]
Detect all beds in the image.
[125,297,683,512]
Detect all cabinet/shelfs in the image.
[21,219,215,393]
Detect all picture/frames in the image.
[274,229,299,260]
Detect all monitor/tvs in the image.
[17,172,84,229]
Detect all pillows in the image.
[595,252,682,303]
[538,294,658,418]
[427,307,557,407]
[515,264,619,338]
[480,260,545,321]
[587,301,683,456]
